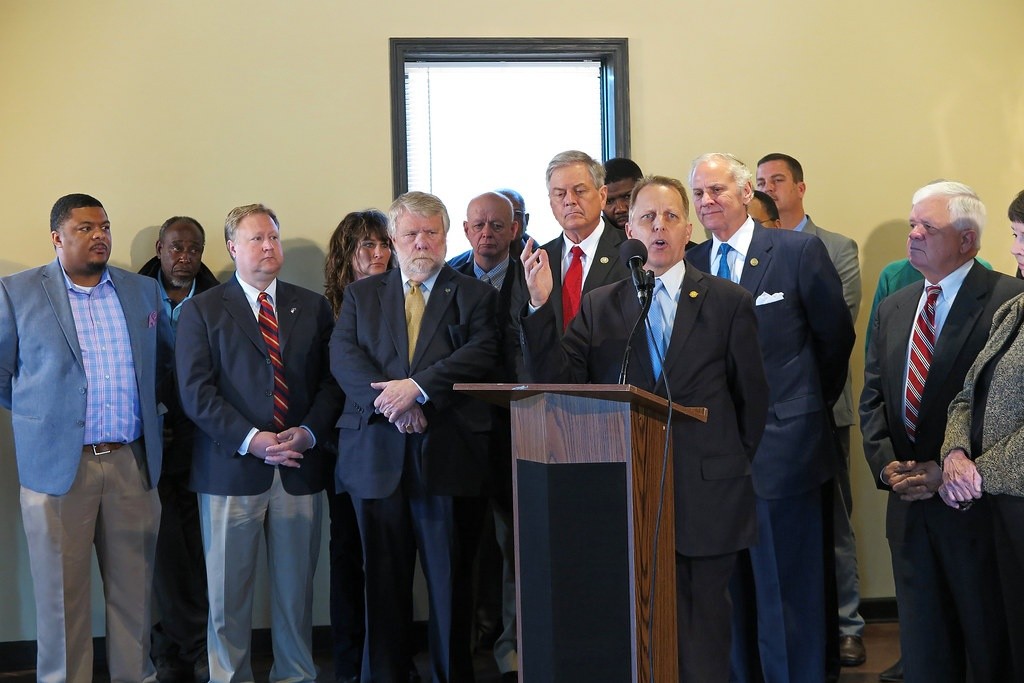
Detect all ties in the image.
[562,246,584,331]
[646,278,665,382]
[479,274,490,283]
[257,291,288,432]
[905,285,943,442]
[716,243,732,281]
[404,280,425,367]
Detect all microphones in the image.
[620,238,648,309]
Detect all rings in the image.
[405,425,411,430]
[385,411,390,416]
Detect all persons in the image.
[324,210,397,683]
[0,193,184,683]
[175,203,346,683]
[494,150,632,683]
[519,175,771,683]
[857,182,1024,683]
[685,153,856,683]
[446,188,539,647]
[602,158,643,231]
[136,216,221,683]
[330,191,499,683]
[747,153,867,683]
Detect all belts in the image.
[83,441,123,456]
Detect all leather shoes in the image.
[840,634,867,666]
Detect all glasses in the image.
[752,217,771,225]
